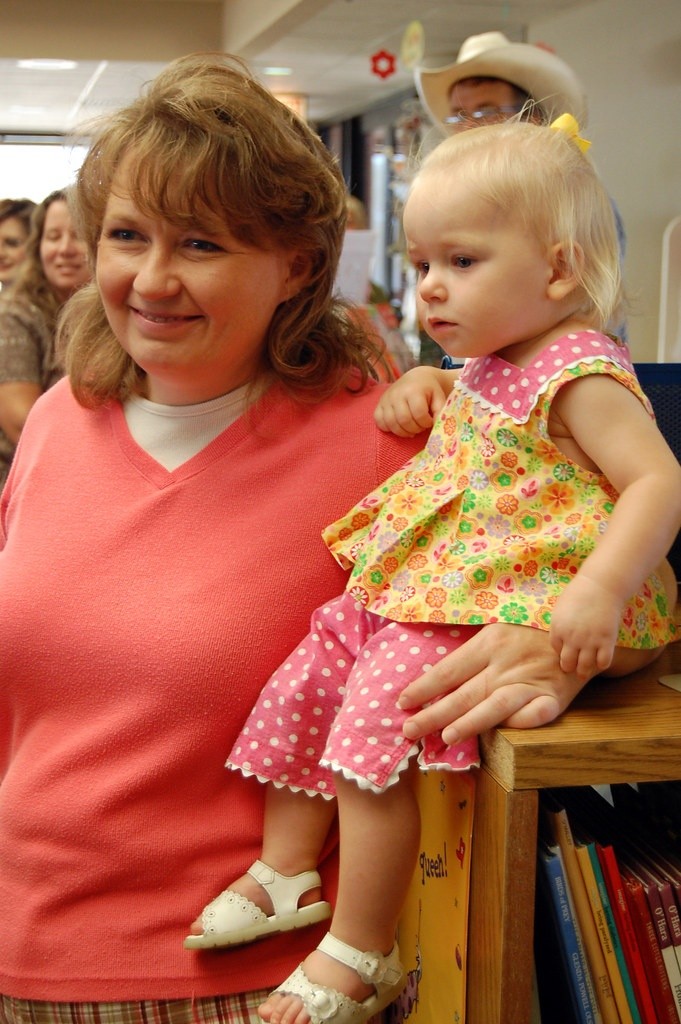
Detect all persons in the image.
[412,33,628,370]
[0,52,677,1024]
[0,197,38,291]
[0,188,96,491]
[181,112,681,1024]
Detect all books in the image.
[539,807,681,1024]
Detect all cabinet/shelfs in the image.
[394,677,681,1024]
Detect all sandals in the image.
[261,931,407,1024]
[182,859,330,950]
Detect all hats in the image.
[416,30,587,137]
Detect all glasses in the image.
[445,106,532,130]
